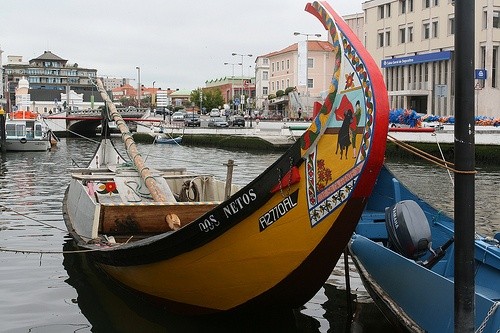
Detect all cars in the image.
[172,109,187,121]
[208,118,229,128]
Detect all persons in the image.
[269,110,273,118]
[298,108,302,118]
[226,108,230,121]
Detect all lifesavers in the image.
[20,138,27,144]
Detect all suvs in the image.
[227,116,245,127]
[156,108,173,116]
[184,113,201,127]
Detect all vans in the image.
[210,108,220,117]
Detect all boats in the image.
[63,1,390,325]
[342,160,500,333]
[155,134,183,144]
[5,106,51,152]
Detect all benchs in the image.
[70,174,214,180]
[65,166,187,174]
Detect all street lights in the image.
[293,32,322,116]
[136,67,140,112]
[232,52,253,114]
[223,62,242,115]
